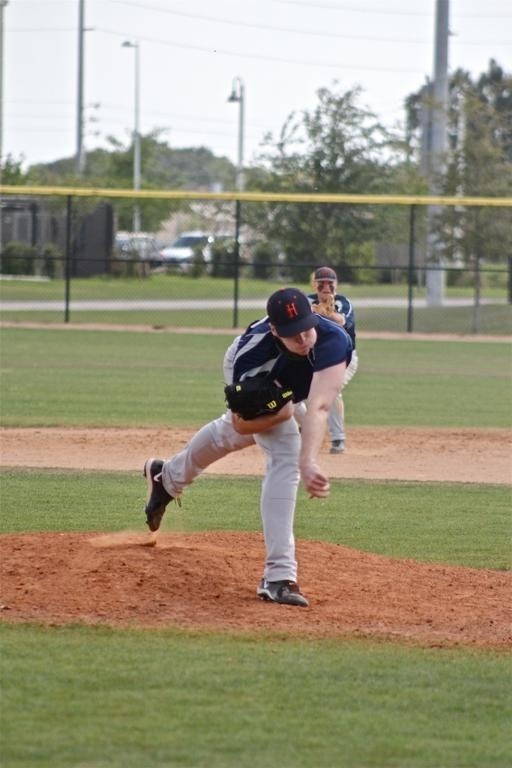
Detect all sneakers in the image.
[143,458,175,532]
[330,440,345,454]
[256,578,309,607]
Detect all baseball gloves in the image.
[312,296,335,319]
[224,371,293,421]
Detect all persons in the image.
[303,267,359,455]
[145,286,352,607]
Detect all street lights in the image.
[121,39,142,231]
[227,75,246,168]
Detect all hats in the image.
[267,287,320,337]
[313,267,337,282]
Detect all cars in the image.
[160,232,212,262]
